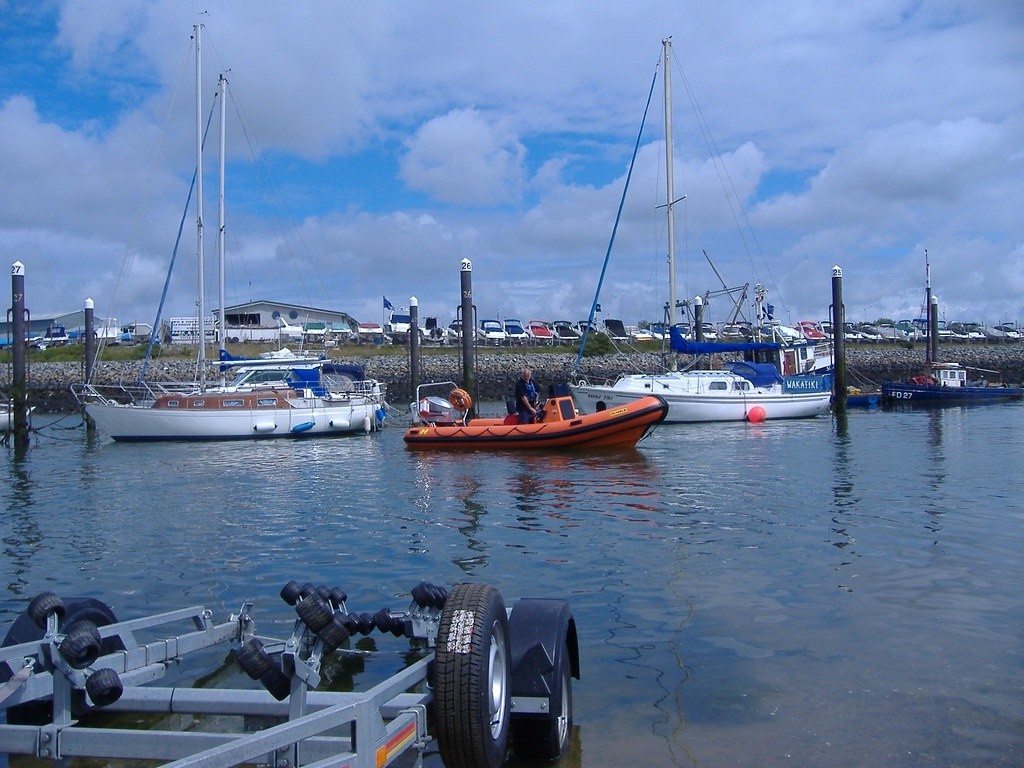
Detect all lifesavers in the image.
[448,389,473,412]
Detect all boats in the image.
[401,380,671,451]
[0,401,37,433]
[274,312,1024,343]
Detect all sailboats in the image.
[568,33,833,419]
[68,21,386,439]
[881,247,1024,398]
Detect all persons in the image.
[515,368,538,425]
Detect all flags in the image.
[383,295,395,311]
[767,303,774,315]
